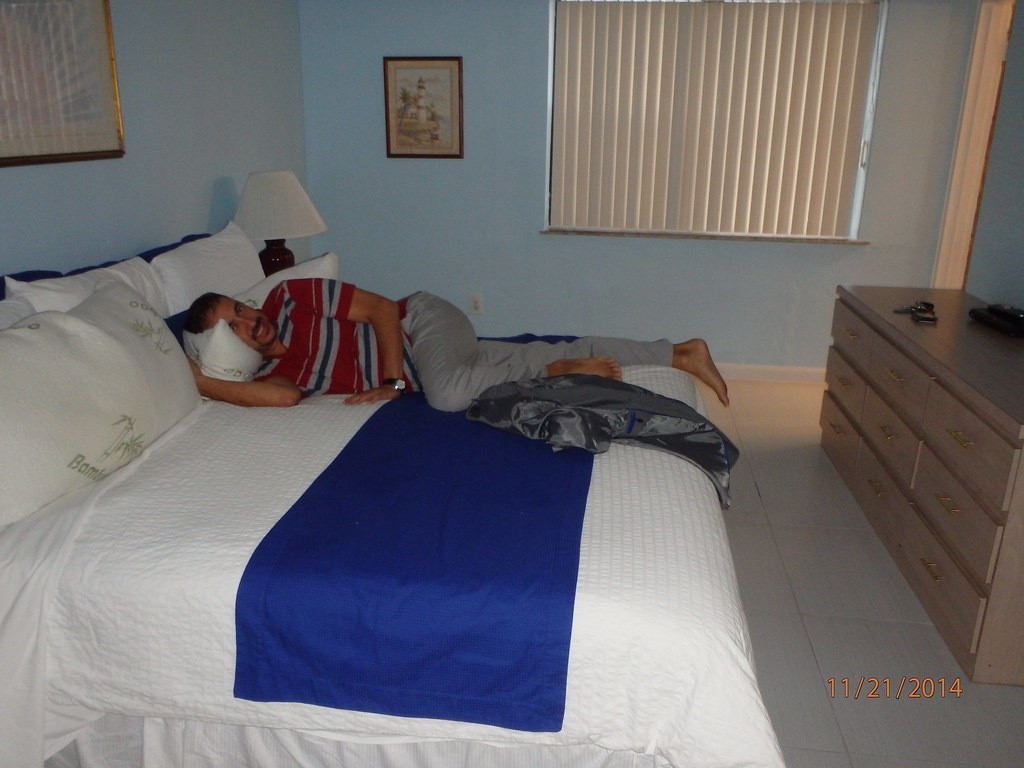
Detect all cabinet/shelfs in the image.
[818,284,1024,688]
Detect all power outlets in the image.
[469,292,484,316]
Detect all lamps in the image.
[235,169,328,279]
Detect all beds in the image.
[0,219,795,768]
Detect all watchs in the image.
[381,377,407,393]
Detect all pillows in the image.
[0,255,172,331]
[0,277,204,534]
[150,220,269,320]
[182,251,340,402]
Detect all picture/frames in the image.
[0,0,127,169]
[384,56,465,160]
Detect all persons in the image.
[174,278,730,408]
